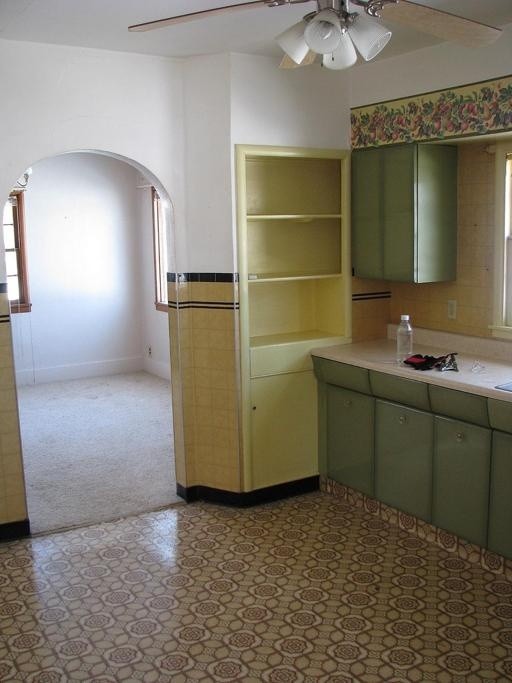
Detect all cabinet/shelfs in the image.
[238,143,351,491]
[319,356,373,500]
[369,371,487,552]
[487,399,512,558]
[351,144,458,285]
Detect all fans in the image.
[127,0,504,69]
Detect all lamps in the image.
[273,7,392,68]
[16,166,33,186]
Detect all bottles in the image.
[395,313,414,364]
